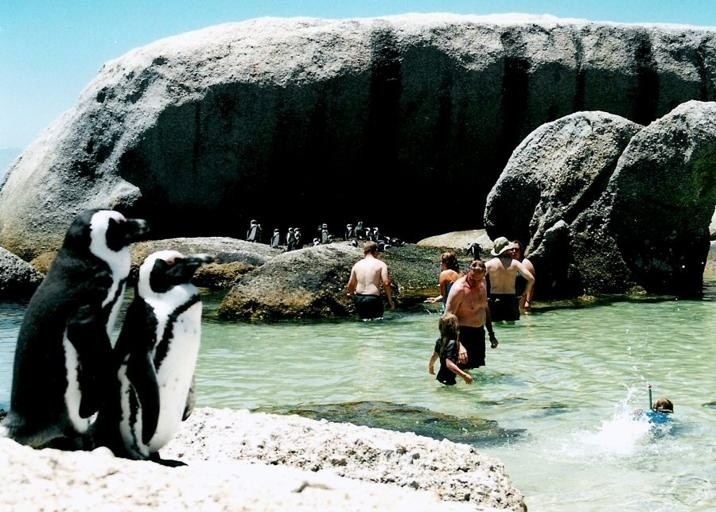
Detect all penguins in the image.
[111,250,216,466]
[269,226,305,252]
[313,220,406,250]
[2,209,151,452]
[464,243,482,261]
[245,219,262,242]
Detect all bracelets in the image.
[488,331,494,336]
[526,299,531,305]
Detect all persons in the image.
[509,240,536,315]
[443,260,498,370]
[429,312,474,385]
[346,240,395,318]
[645,398,674,424]
[483,236,535,321]
[427,251,462,307]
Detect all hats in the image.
[654,397,675,413]
[489,236,519,257]
